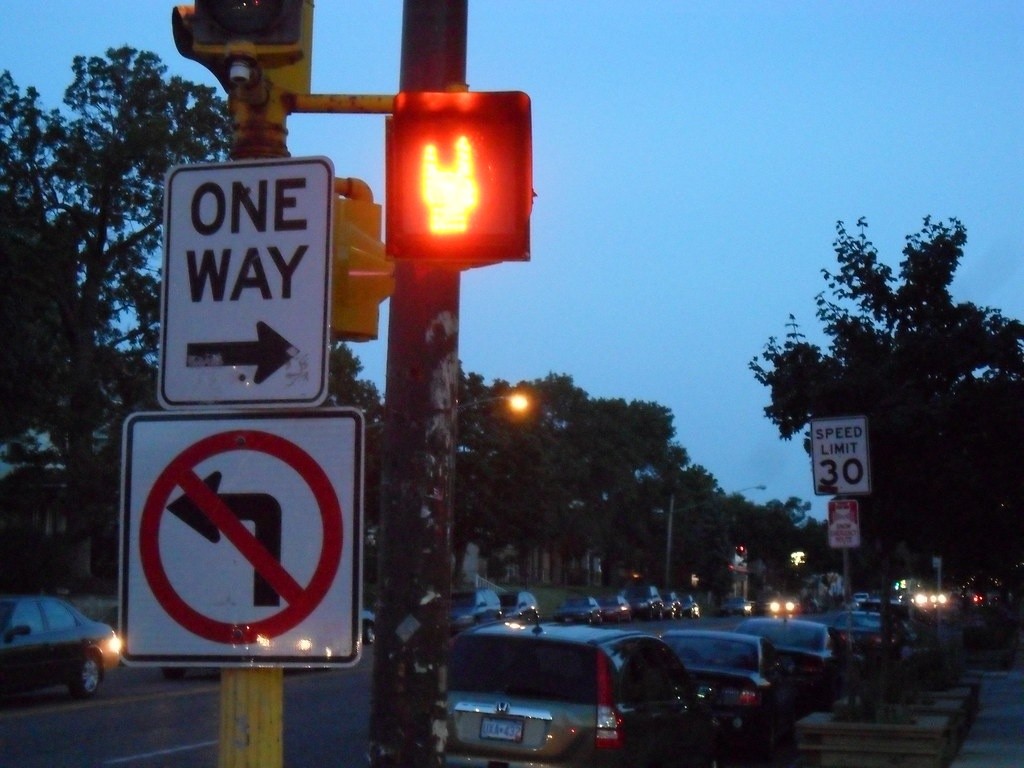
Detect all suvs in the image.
[428,615,726,767]
[448,583,502,635]
[620,584,666,621]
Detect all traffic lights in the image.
[386,92,535,261]
[167,0,311,92]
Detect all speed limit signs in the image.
[812,417,873,499]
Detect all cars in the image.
[0,593,121,705]
[729,611,845,716]
[652,628,798,767]
[718,596,756,618]
[828,606,915,680]
[553,595,604,626]
[758,576,814,617]
[658,590,685,623]
[678,592,702,619]
[495,589,540,624]
[823,592,924,673]
[599,592,631,625]
